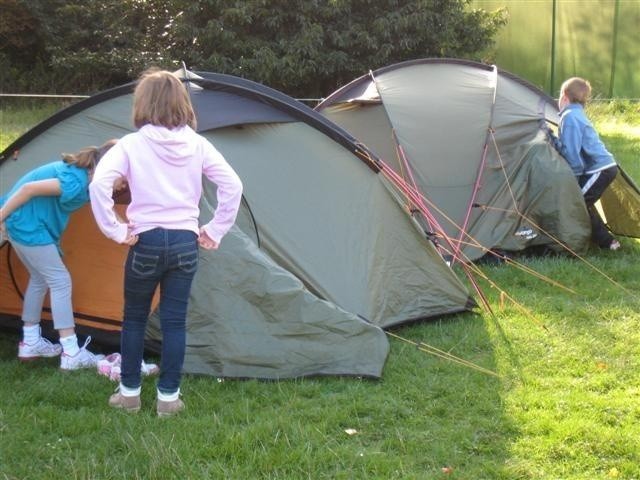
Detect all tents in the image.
[0,60,482,380]
[309,55,639,264]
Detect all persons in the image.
[547,76,623,252]
[88,68,244,416]
[0,138,130,370]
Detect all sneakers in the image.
[610,239,620,250]
[19,338,62,359]
[60,347,104,369]
[156,400,185,415]
[108,393,140,410]
[98,353,157,381]
